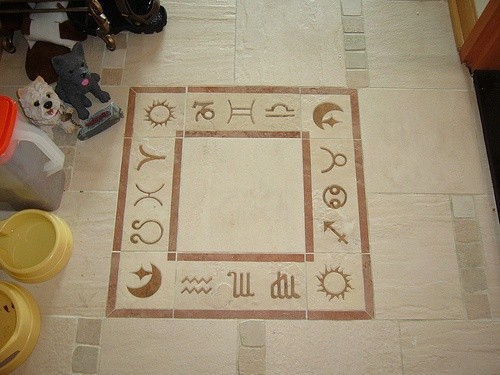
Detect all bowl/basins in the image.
[0,280,41,375]
[0,208,73,284]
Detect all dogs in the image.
[18,75,76,140]
[50,42,111,120]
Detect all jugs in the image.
[0,95,67,212]
